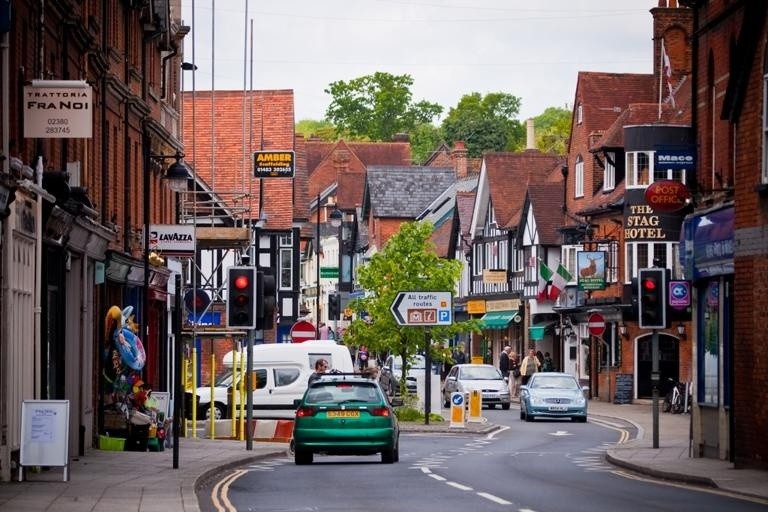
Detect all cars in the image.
[378,354,437,396]
[520,371,590,423]
[441,363,510,409]
[294,372,404,456]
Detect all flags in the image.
[549,263,572,304]
[535,261,554,304]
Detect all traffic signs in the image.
[389,290,453,327]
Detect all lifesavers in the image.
[103,306,146,370]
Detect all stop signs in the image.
[290,321,317,343]
[588,313,606,337]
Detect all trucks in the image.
[185,339,355,421]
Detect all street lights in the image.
[315,193,344,339]
[141,122,193,390]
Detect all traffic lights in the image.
[617,275,638,324]
[638,267,666,330]
[256,270,276,330]
[667,270,693,329]
[226,266,256,329]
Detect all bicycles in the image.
[667,383,690,414]
[661,377,686,414]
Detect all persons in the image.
[295,322,556,402]
